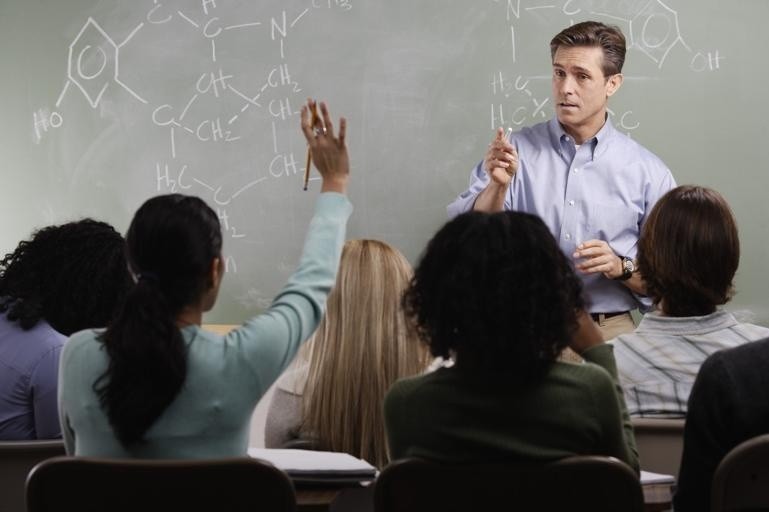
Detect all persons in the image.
[378,207,644,480]
[56,98,353,464]
[580,185,769,418]
[450,19,681,366]
[1,217,139,441]
[255,238,440,473]
[673,337,769,512]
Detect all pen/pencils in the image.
[303,100,317,190]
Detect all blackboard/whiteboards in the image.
[0,0,769,338]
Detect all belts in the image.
[588,312,627,321]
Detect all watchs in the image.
[614,254,635,283]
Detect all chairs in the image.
[0,434,769,512]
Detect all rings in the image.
[313,126,326,137]
